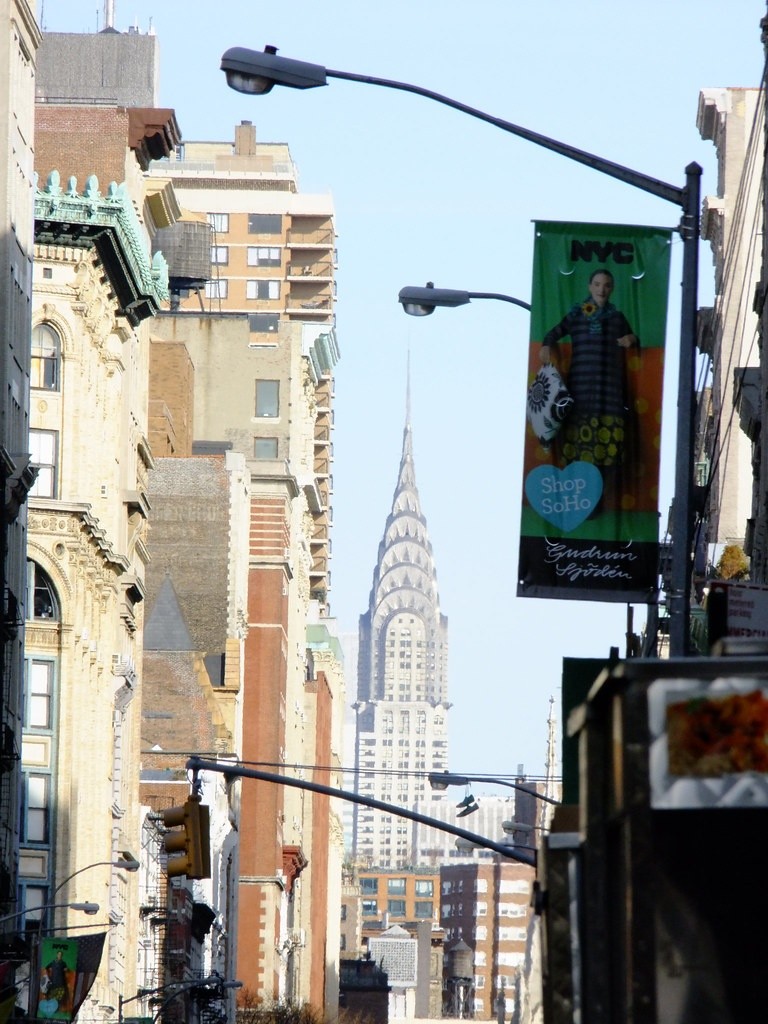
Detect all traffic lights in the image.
[162,802,212,880]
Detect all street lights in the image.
[27,858,139,1024]
[397,281,657,662]
[223,41,703,657]
[118,976,220,1024]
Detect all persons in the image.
[45,952,72,1008]
[540,269,641,519]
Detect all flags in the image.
[65,932,107,1022]
